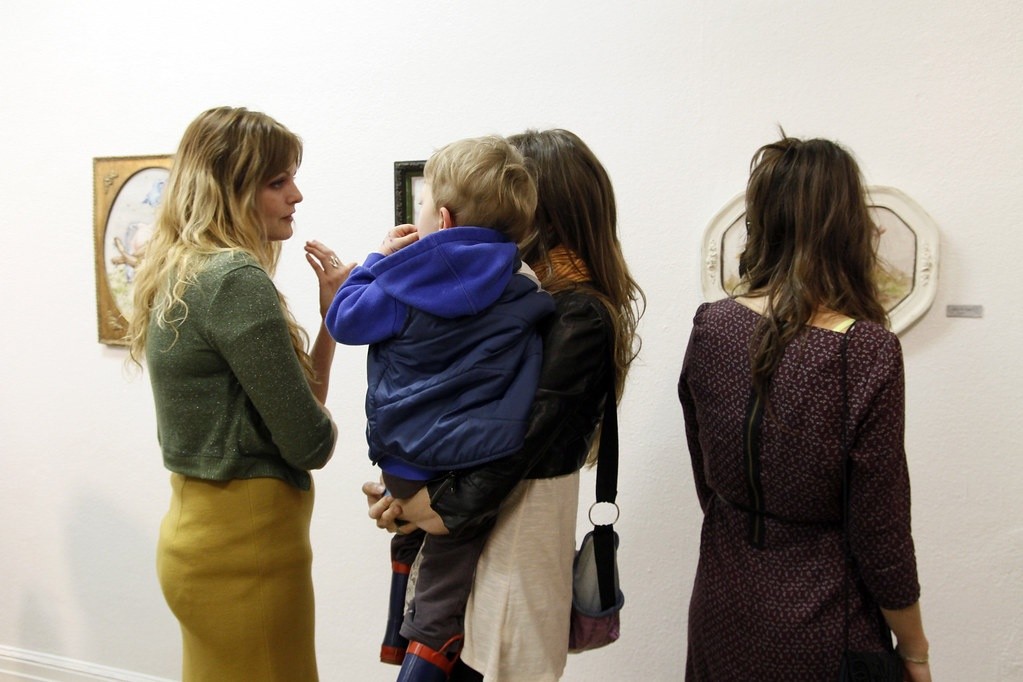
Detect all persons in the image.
[134,106,358,682]
[677,135,931,681]
[323,136,555,682]
[363,127,632,681]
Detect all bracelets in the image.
[895,645,929,664]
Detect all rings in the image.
[328,254,340,267]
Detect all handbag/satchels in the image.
[836,649,903,682]
[568,524,624,654]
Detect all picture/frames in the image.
[701,187,940,334]
[92,152,189,347]
[393,162,445,227]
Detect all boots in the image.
[379,553,409,665]
[396,635,484,682]
